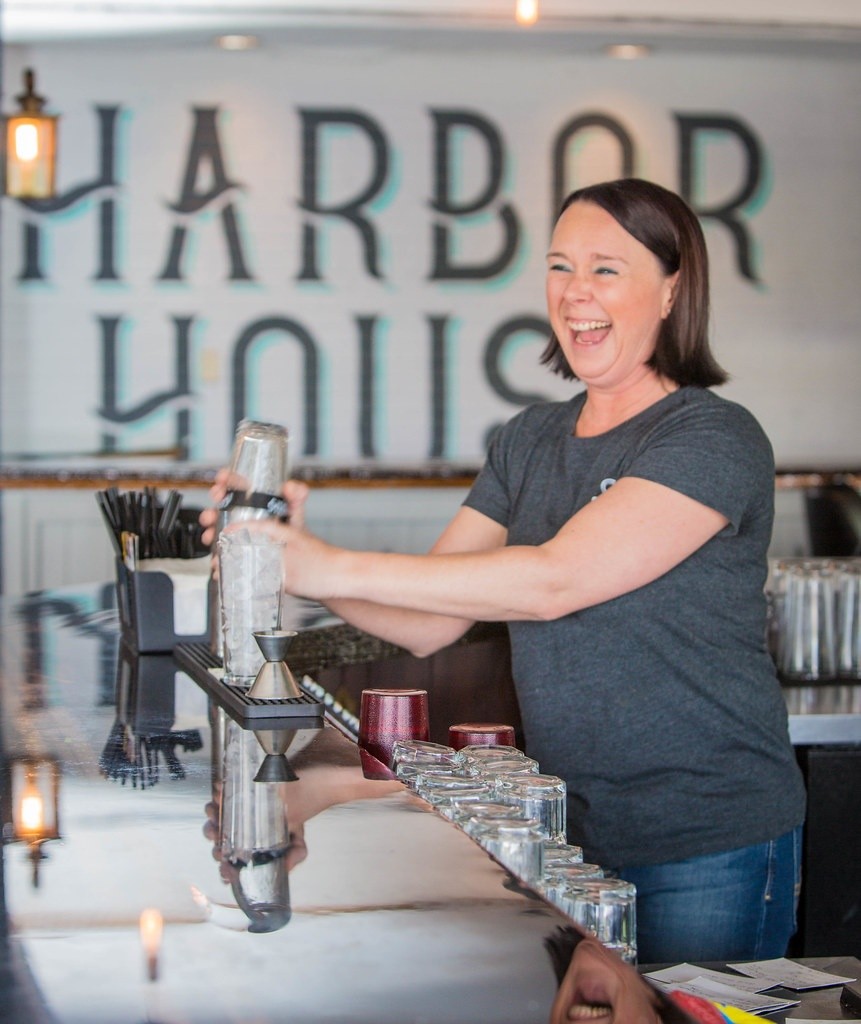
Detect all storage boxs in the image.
[114,557,219,653]
[113,638,208,732]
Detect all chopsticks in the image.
[96,483,184,561]
[97,723,177,790]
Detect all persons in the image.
[200,175,806,970]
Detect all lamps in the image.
[0,66,60,203]
[8,749,60,890]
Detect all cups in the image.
[206,540,284,659]
[357,688,429,772]
[446,722,516,758]
[217,537,285,689]
[220,847,292,936]
[208,702,233,820]
[211,420,292,550]
[764,556,860,682]
[387,735,639,990]
[221,718,290,856]
